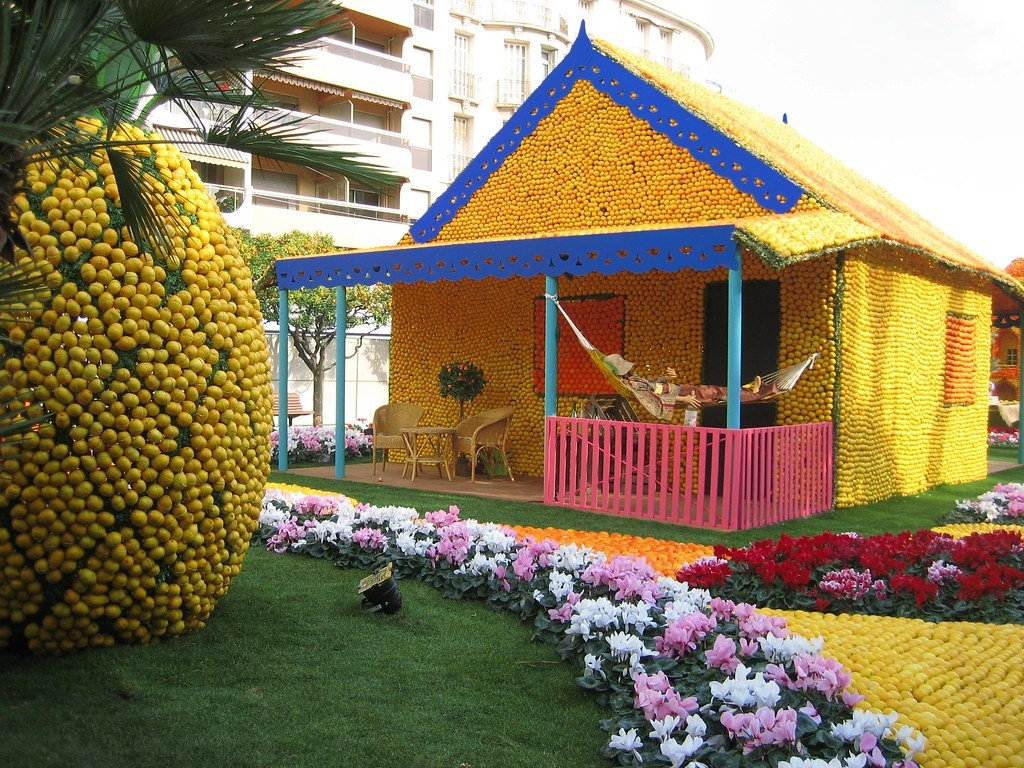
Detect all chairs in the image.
[452,408,516,483]
[373,402,424,477]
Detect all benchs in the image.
[272,393,314,426]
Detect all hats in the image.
[603,354,635,375]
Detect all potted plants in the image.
[438,360,486,475]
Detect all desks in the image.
[399,426,455,482]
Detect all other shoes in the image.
[752,375,761,393]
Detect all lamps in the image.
[358,562,402,616]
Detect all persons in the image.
[603,352,782,423]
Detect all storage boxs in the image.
[555,392,657,495]
